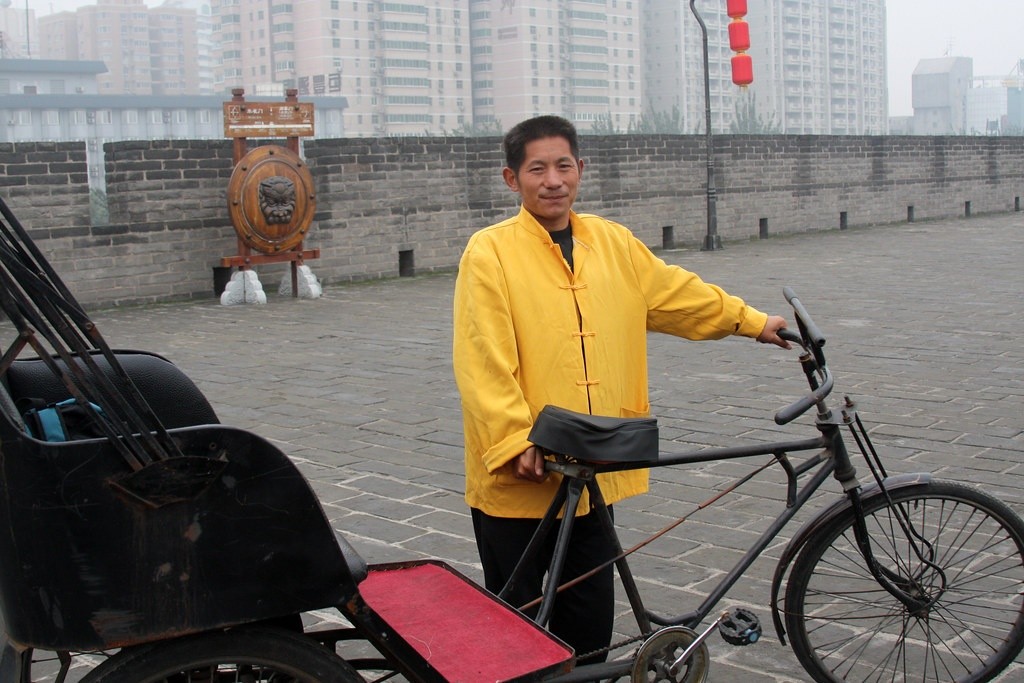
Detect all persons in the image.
[451,115,790,682]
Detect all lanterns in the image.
[727,0,753,91]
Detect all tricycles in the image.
[0,183,1024,683]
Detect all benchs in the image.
[0,348,368,652]
